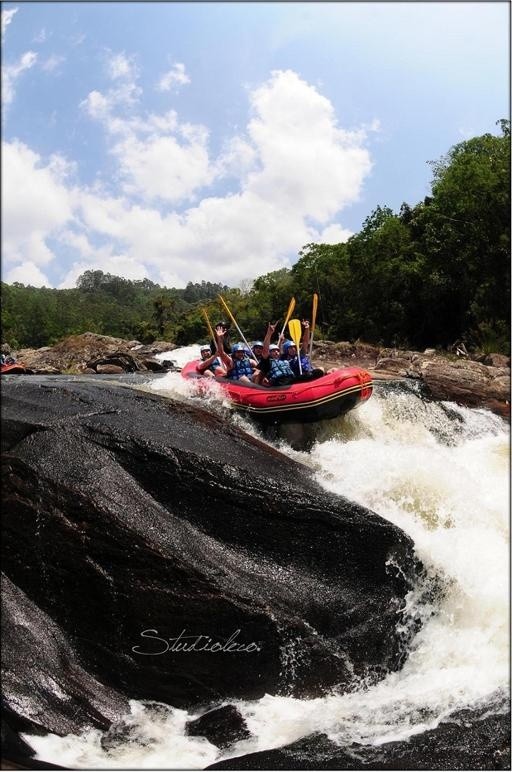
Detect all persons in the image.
[196,345,226,378]
[215,326,269,385]
[277,318,324,385]
[241,340,264,365]
[208,321,237,355]
[260,319,296,386]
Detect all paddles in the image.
[200,307,219,352]
[308,293,318,361]
[216,294,259,364]
[277,297,296,347]
[288,319,303,376]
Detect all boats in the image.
[183,357,373,424]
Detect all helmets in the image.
[216,321,227,327]
[200,344,211,352]
[231,339,296,354]
